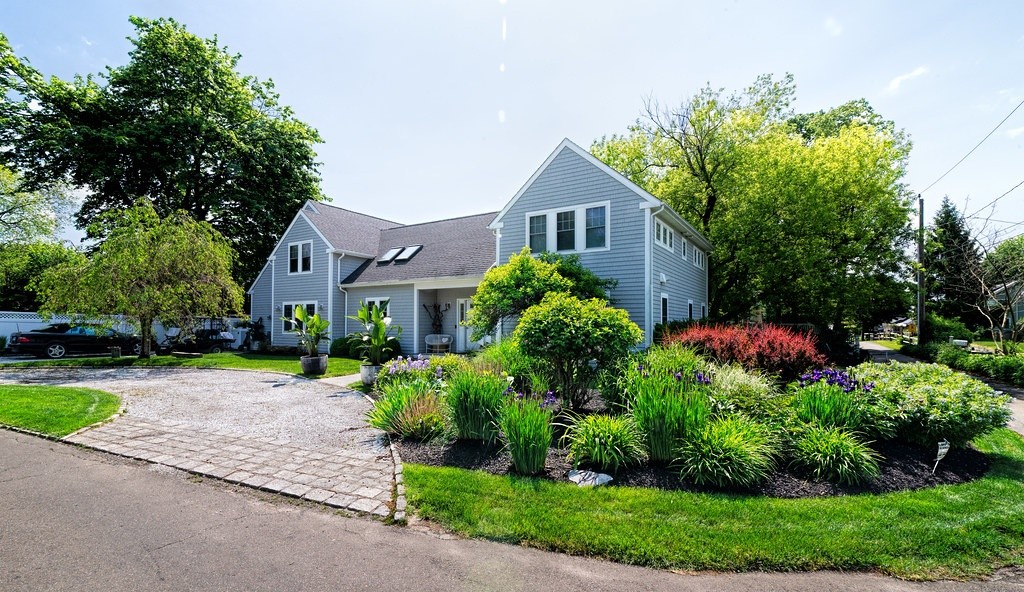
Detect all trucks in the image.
[155,316,236,356]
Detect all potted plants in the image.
[282,305,332,375]
[346,301,403,386]
[249,323,266,350]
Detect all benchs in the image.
[185,329,211,343]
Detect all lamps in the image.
[276,305,281,311]
[319,303,324,311]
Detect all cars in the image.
[5,322,159,360]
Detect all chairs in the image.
[78,328,85,335]
[424,334,454,353]
[72,328,77,331]
